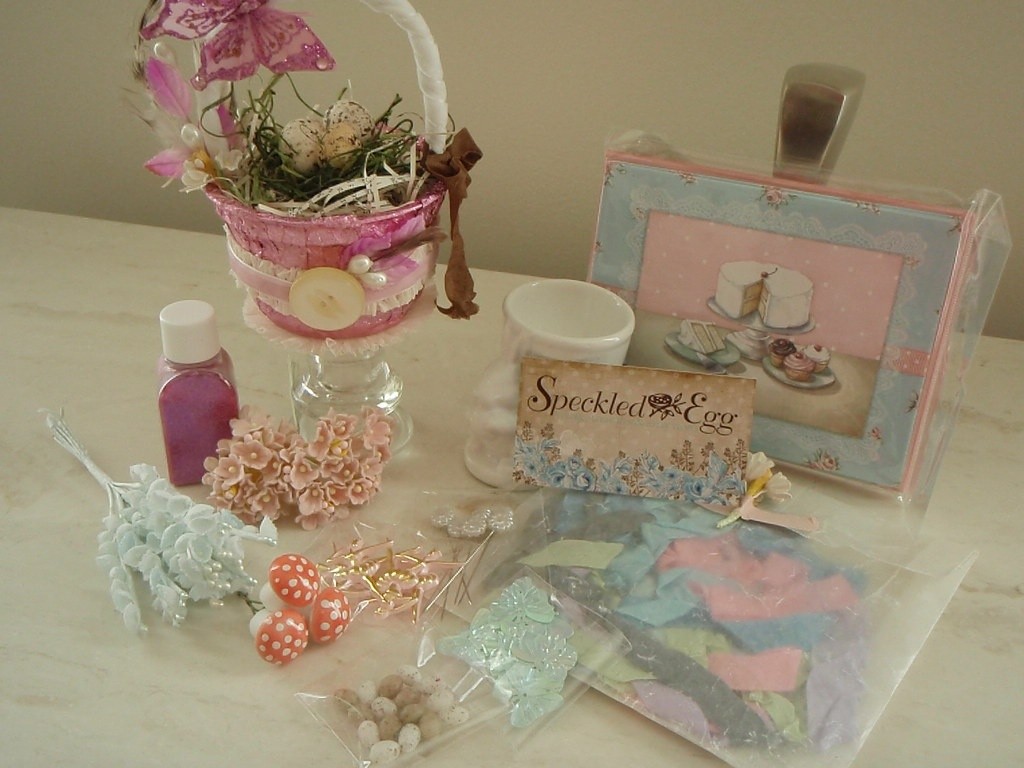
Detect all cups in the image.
[460,278,636,488]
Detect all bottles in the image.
[158,300,240,485]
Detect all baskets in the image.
[195,0,446,338]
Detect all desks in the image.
[2,208,1023,768]
